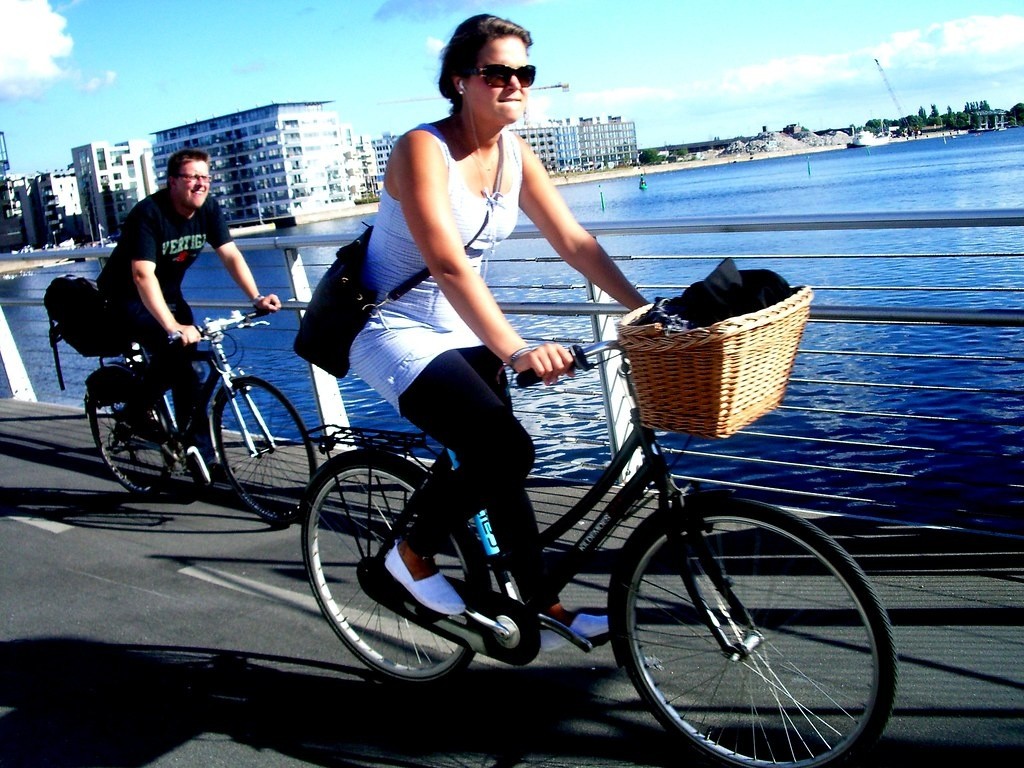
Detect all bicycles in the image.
[298,339,902,768]
[82,309,321,529]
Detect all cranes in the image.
[378,81,572,128]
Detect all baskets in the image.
[614,286,815,439]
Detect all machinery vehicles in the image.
[873,57,922,136]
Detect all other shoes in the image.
[191,462,235,483]
[384,539,466,615]
[540,612,609,651]
[123,403,167,443]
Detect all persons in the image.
[102,149,284,485]
[349,14,651,653]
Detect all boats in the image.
[638,173,648,189]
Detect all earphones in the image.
[459,80,464,89]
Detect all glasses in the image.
[472,64,536,88]
[175,172,212,183]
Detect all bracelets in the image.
[252,294,263,304]
[509,344,538,367]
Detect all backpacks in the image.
[42,273,122,390]
